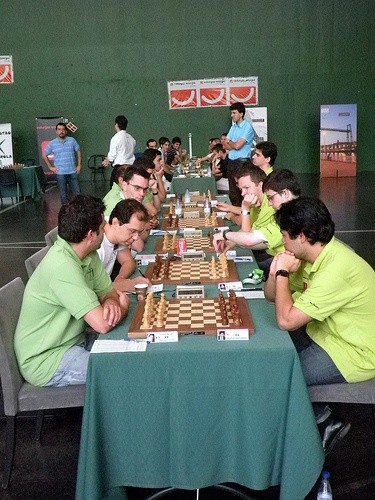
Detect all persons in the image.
[13,195,130,390]
[41,123,82,203]
[96,198,152,295]
[264,196,375,385]
[212,168,300,292]
[101,114,136,189]
[99,136,188,228]
[105,167,152,254]
[193,132,228,190]
[234,166,277,232]
[222,101,255,207]
[214,140,279,225]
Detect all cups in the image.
[135,283,148,302]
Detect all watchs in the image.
[222,229,232,240]
[275,269,289,278]
[240,210,250,215]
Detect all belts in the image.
[239,158,250,162]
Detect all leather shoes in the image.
[321,418,351,456]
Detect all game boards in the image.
[127,291,255,339]
[2,163,25,168]
[143,253,240,285]
[162,211,225,231]
[163,189,231,219]
[154,229,234,254]
[181,155,209,177]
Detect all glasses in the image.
[267,188,290,201]
[118,219,145,236]
[125,181,150,193]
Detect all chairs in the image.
[307,381,375,407]
[19,246,49,279]
[0,169,24,205]
[21,159,35,166]
[0,277,84,490]
[44,226,60,248]
[87,154,107,184]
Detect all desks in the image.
[76,195,325,500]
[171,155,217,197]
[0,165,43,206]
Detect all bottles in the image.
[204,198,211,216]
[315,471,333,500]
[184,189,190,204]
[175,198,183,216]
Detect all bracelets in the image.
[226,139,231,144]
[153,191,159,196]
